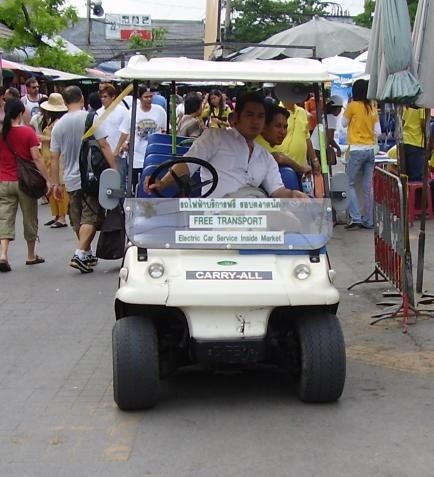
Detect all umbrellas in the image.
[230,12,371,58]
[412,0,434,293]
[368,0,421,303]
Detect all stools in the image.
[406,182,432,227]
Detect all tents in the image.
[0,59,120,83]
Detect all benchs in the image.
[136,134,303,210]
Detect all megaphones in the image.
[93,5,104,16]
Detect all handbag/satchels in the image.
[314,173,325,199]
[327,147,337,165]
[21,160,47,199]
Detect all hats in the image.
[40,93,69,111]
[328,95,343,106]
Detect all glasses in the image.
[28,87,40,89]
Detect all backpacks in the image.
[79,113,113,194]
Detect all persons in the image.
[0,97,52,272]
[0,72,434,228]
[92,84,129,190]
[144,94,310,199]
[50,87,117,272]
[113,85,169,195]
[342,79,378,228]
[311,95,345,165]
[254,103,312,177]
[277,100,322,179]
[177,93,206,138]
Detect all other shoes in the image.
[345,222,357,229]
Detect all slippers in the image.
[0,262,10,272]
[44,220,54,225]
[26,255,45,264]
[51,221,67,228]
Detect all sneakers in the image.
[85,255,97,265]
[70,255,92,273]
